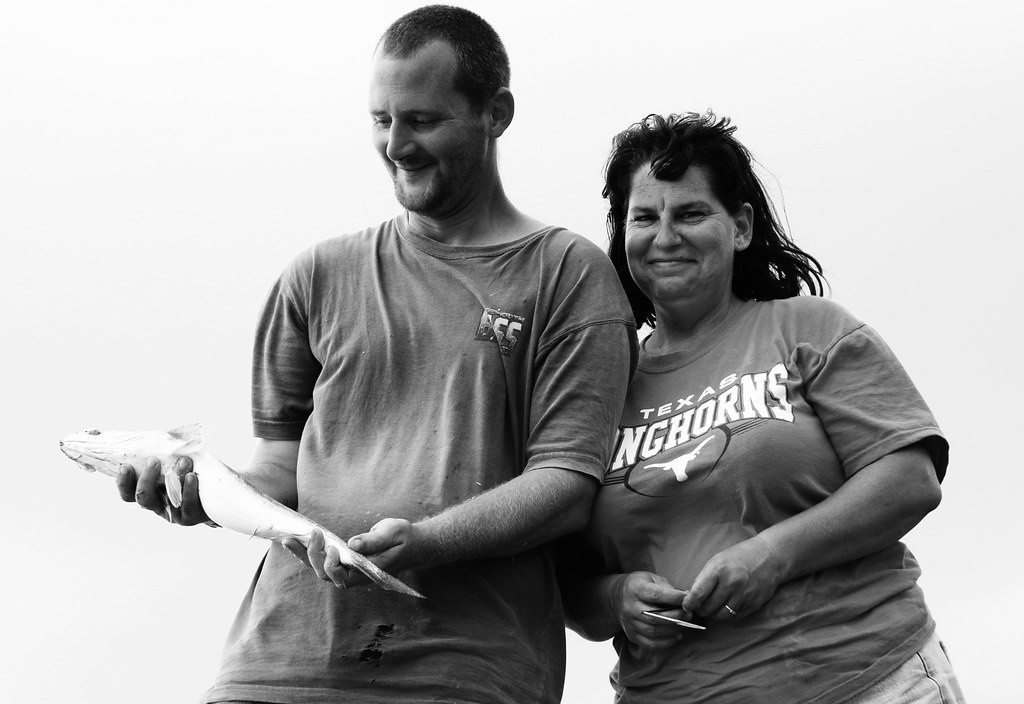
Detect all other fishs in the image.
[58,423,425,598]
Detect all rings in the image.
[725,604,737,616]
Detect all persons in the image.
[561,113,966,703]
[115,5,640,704]
